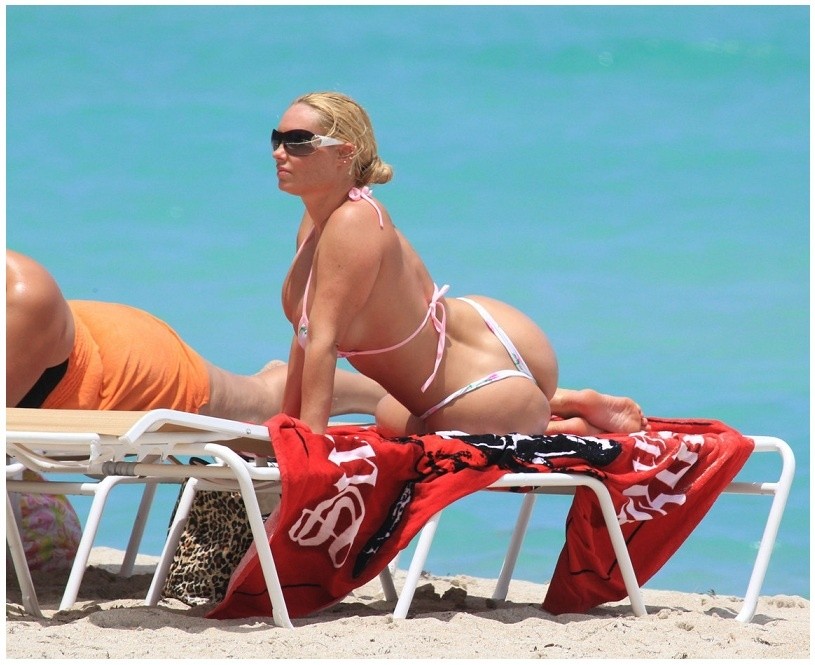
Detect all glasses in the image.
[271,128,344,157]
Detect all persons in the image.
[5,249,389,429]
[269,92,654,438]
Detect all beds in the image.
[5,406,794,629]
